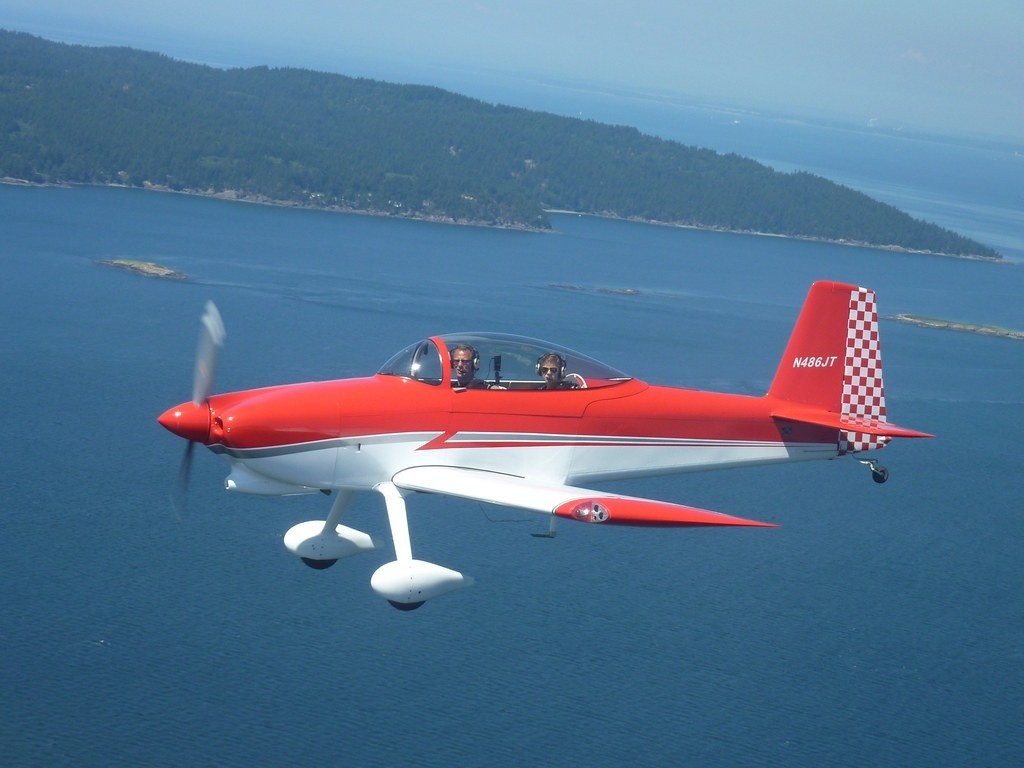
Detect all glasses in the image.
[452,359,471,365]
[540,367,557,373]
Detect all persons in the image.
[449,344,488,389]
[536,352,581,390]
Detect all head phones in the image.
[449,349,480,370]
[535,354,567,379]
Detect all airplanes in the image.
[156,282,936,612]
